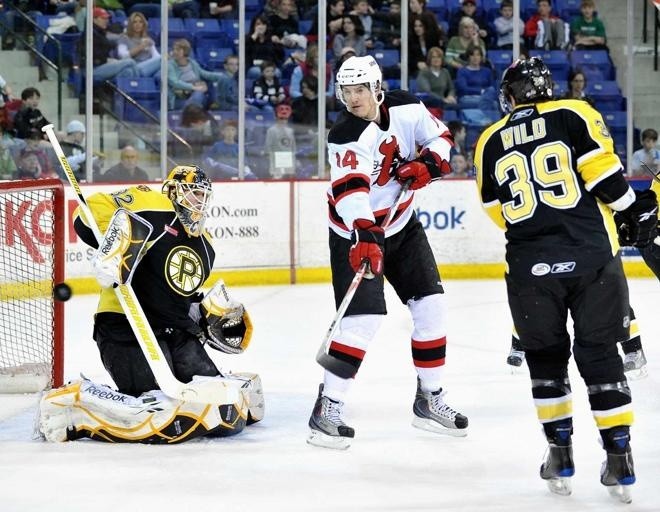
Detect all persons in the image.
[307,54,469,438]
[37,165,267,444]
[618,213,660,282]
[473,55,657,486]
[632,129,660,176]
[649,171,660,236]
[1,0,607,181]
[505,305,647,372]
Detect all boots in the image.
[539,427,575,482]
[307,386,357,439]
[411,376,470,429]
[599,431,636,488]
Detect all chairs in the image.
[0,0,642,181]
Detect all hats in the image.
[92,6,110,20]
[65,120,86,134]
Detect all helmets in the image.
[333,53,386,122]
[498,56,556,113]
[160,163,215,239]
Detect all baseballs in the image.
[53,283,71,301]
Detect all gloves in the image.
[615,189,659,250]
[347,217,385,279]
[395,148,453,196]
